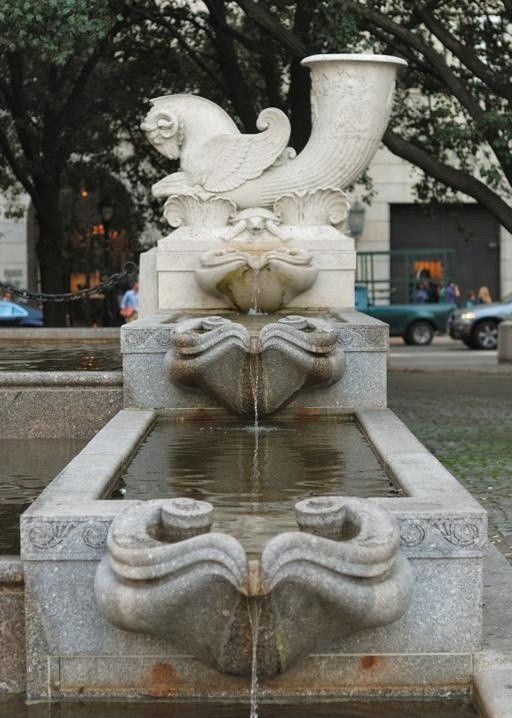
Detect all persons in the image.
[409,274,491,308]
[1,279,139,328]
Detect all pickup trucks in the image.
[355,283,456,345]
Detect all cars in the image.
[446,291,512,348]
